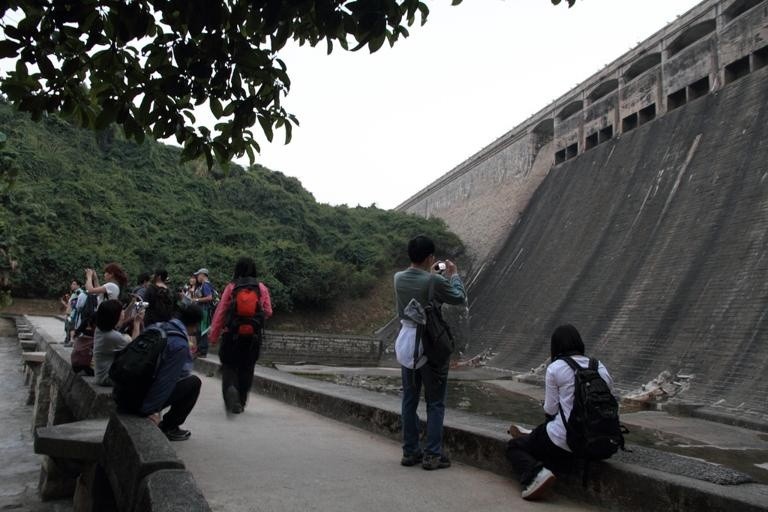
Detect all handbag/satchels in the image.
[202,282,220,306]
[95,299,122,332]
[413,274,454,369]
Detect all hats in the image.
[193,269,209,275]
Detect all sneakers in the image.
[401,448,423,466]
[521,467,557,499]
[197,353,206,357]
[227,387,238,407]
[423,449,451,469]
[232,403,244,412]
[510,425,533,438]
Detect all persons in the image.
[208,256,274,417]
[60,263,219,389]
[394,233,467,471]
[107,302,204,440]
[506,322,619,500]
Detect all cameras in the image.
[436,262,447,271]
[135,301,149,310]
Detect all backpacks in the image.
[109,322,187,393]
[559,356,624,461]
[119,291,143,325]
[229,278,264,335]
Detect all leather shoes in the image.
[158,420,190,439]
